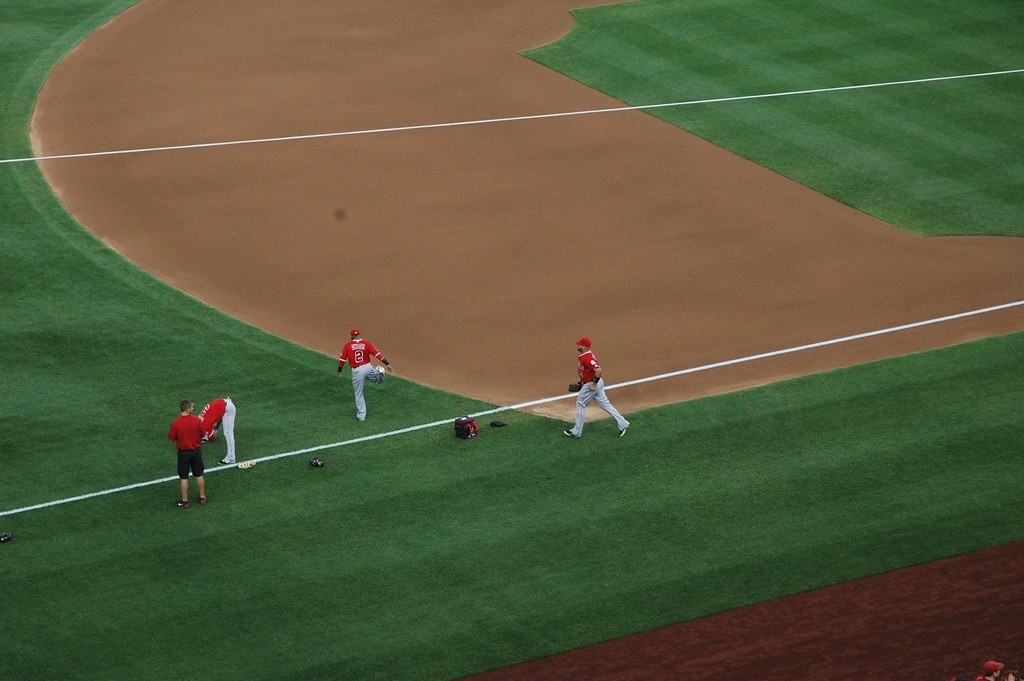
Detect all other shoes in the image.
[377,365,386,375]
[359,417,365,421]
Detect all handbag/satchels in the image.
[454,415,479,439]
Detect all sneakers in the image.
[199,496,207,504]
[217,458,235,464]
[564,429,580,438]
[619,426,627,437]
[176,499,190,508]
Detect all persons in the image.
[564,337,630,436]
[337,329,393,423]
[198,398,239,462]
[167,401,207,508]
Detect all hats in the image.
[982,660,1004,675]
[351,327,360,340]
[576,336,592,348]
